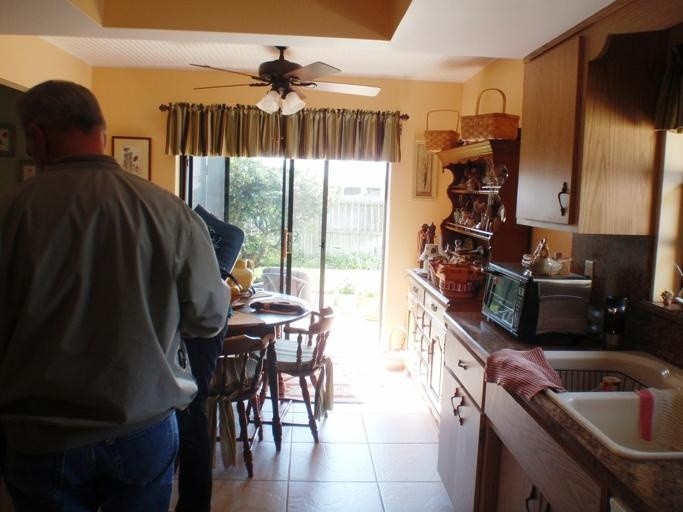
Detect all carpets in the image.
[285,381,364,403]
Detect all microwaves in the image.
[480,259,592,342]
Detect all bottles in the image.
[604,294,633,348]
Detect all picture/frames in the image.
[21,160,37,182]
[0,123,16,157]
[112,135,152,181]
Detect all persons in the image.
[176,295,232,511]
[1,78,229,510]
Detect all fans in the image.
[191,46,382,97]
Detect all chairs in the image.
[217,305,337,480]
[262,267,308,300]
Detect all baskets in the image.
[552,369,648,393]
[460,89,520,142]
[423,109,460,152]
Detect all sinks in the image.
[546,351,683,394]
[552,395,683,458]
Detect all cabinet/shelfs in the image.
[515,32,660,235]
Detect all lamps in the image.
[256,82,305,116]
